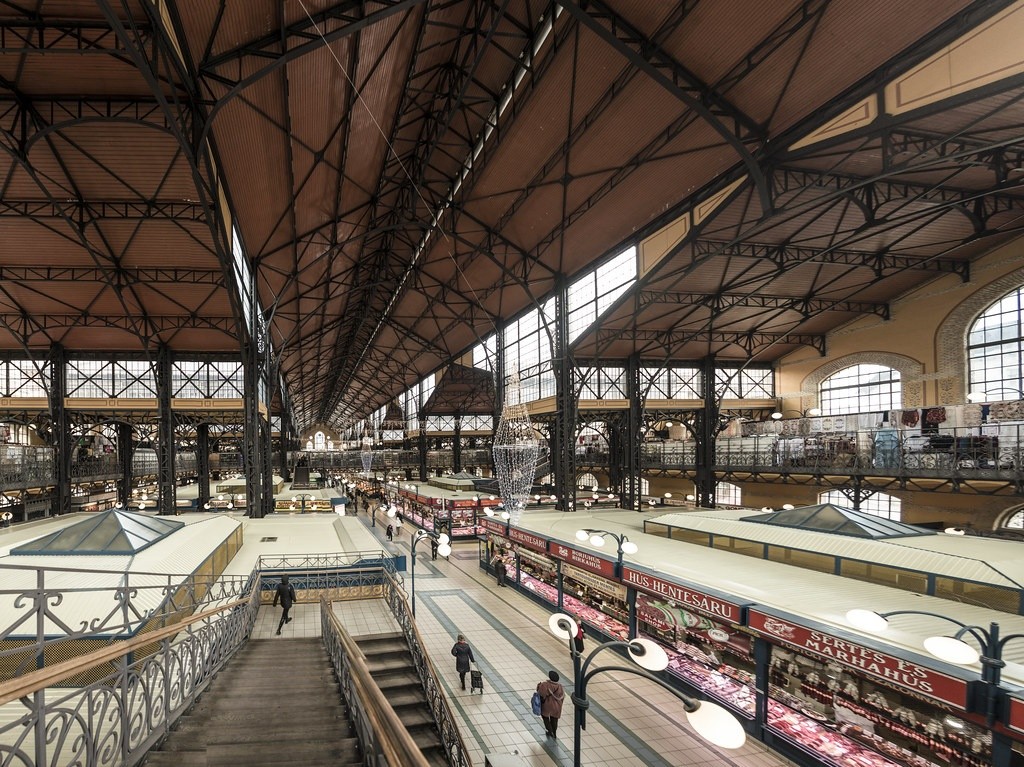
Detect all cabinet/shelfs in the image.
[387,501,905,767]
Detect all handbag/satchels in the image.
[531,693,543,716]
[386,530,390,536]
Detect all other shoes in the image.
[546,731,556,738]
[275,630,281,635]
[284,617,292,624]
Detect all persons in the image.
[451,634,476,690]
[575,445,588,455]
[362,498,366,507]
[396,517,402,535]
[364,502,370,512]
[570,616,585,660]
[272,574,297,635]
[587,442,595,453]
[396,510,404,524]
[495,557,507,587]
[537,670,566,737]
[386,524,393,541]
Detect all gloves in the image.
[471,659,474,662]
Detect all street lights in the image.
[409,528,453,620]
[334,475,355,497]
[545,608,749,767]
[371,503,396,528]
